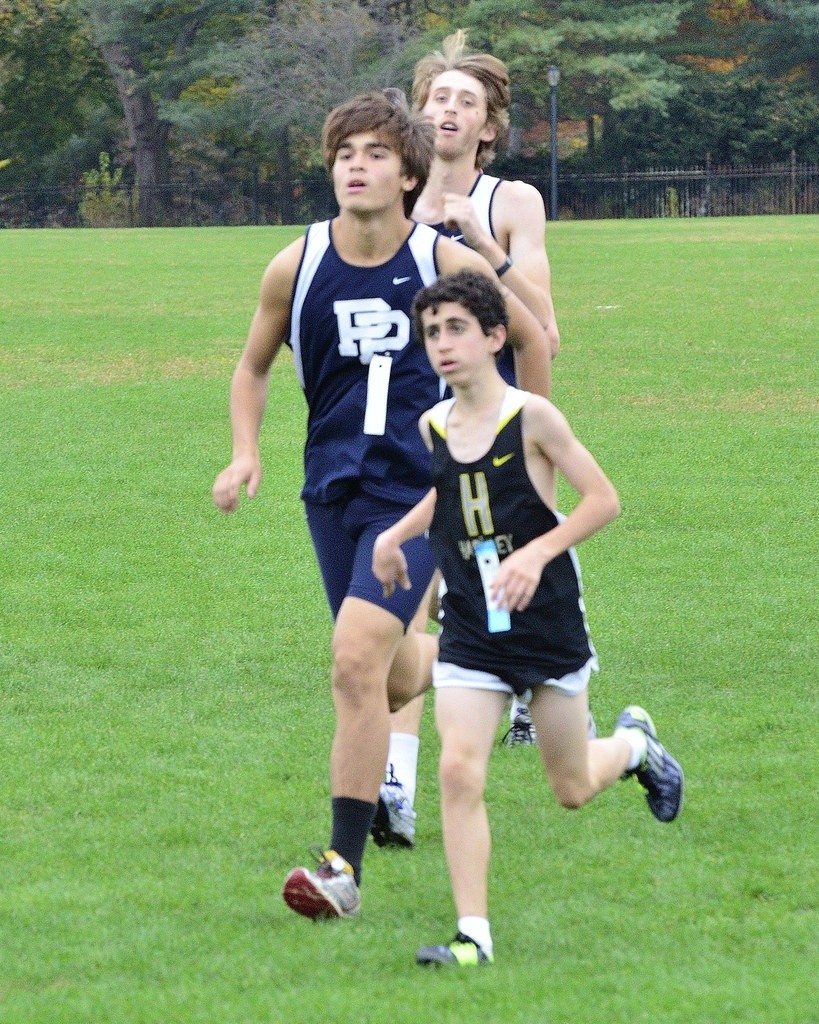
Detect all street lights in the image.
[545,63,561,219]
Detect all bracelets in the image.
[496,256,512,278]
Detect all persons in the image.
[370,266,683,969]
[212,88,549,916]
[367,31,559,852]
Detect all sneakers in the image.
[282,845,364,921]
[502,706,537,748]
[616,704,684,823]
[370,762,416,848]
[415,932,492,969]
